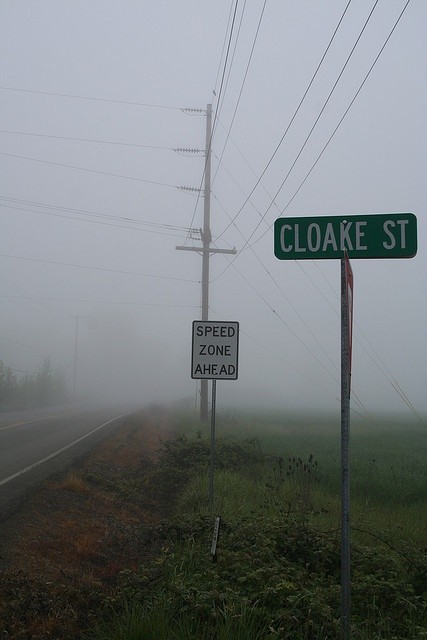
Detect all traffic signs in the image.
[272,212,419,260]
[189,319,240,379]
